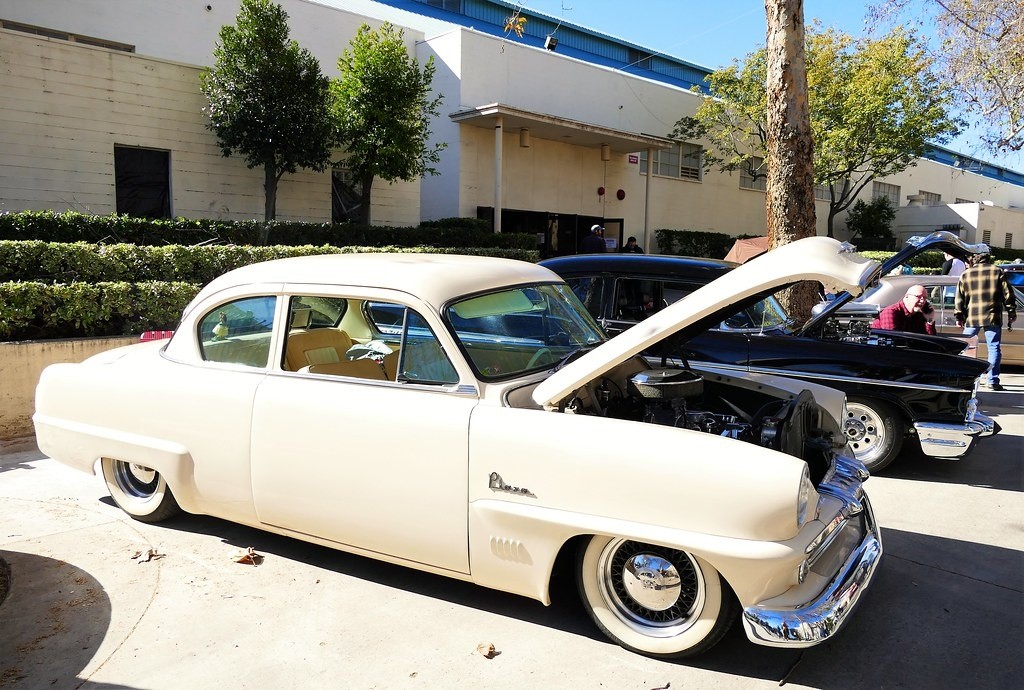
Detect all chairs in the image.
[383,341,458,382]
[296,358,388,381]
[226,342,292,371]
[286,327,354,373]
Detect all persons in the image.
[941,251,966,309]
[887,261,913,276]
[954,245,1018,391]
[870,285,936,335]
[580,225,608,253]
[619,236,643,254]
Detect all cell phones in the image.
[922,301,933,315]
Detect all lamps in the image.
[543,36,558,51]
[520,128,529,147]
[601,143,610,161]
[954,161,960,167]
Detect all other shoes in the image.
[987,384,1004,391]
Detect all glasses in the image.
[908,294,927,301]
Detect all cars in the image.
[812,264,1024,366]
[33,237,884,662]
[365,230,1001,477]
